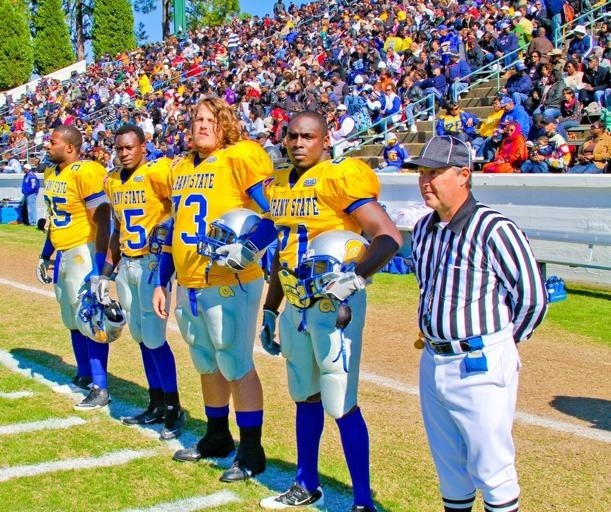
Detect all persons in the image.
[95,124,185,440]
[36,124,112,412]
[150,97,276,481]
[0,0,611,175]
[21,163,40,226]
[260,110,403,512]
[403,135,548,512]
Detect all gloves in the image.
[95,275,112,306]
[257,307,280,355]
[35,258,52,284]
[315,268,363,304]
[213,240,259,273]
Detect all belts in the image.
[427,336,481,359]
[122,254,143,258]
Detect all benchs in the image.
[402,19,611,173]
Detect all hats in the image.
[547,48,564,56]
[497,97,513,107]
[23,163,31,170]
[540,118,556,124]
[514,62,528,71]
[586,55,599,62]
[400,133,471,174]
[574,25,586,35]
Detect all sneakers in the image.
[122,404,163,426]
[220,445,266,482]
[160,401,186,440]
[66,374,94,391]
[174,434,236,461]
[74,383,111,410]
[258,481,324,511]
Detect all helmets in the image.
[194,208,265,260]
[146,216,176,260]
[76,286,126,344]
[295,229,374,304]
